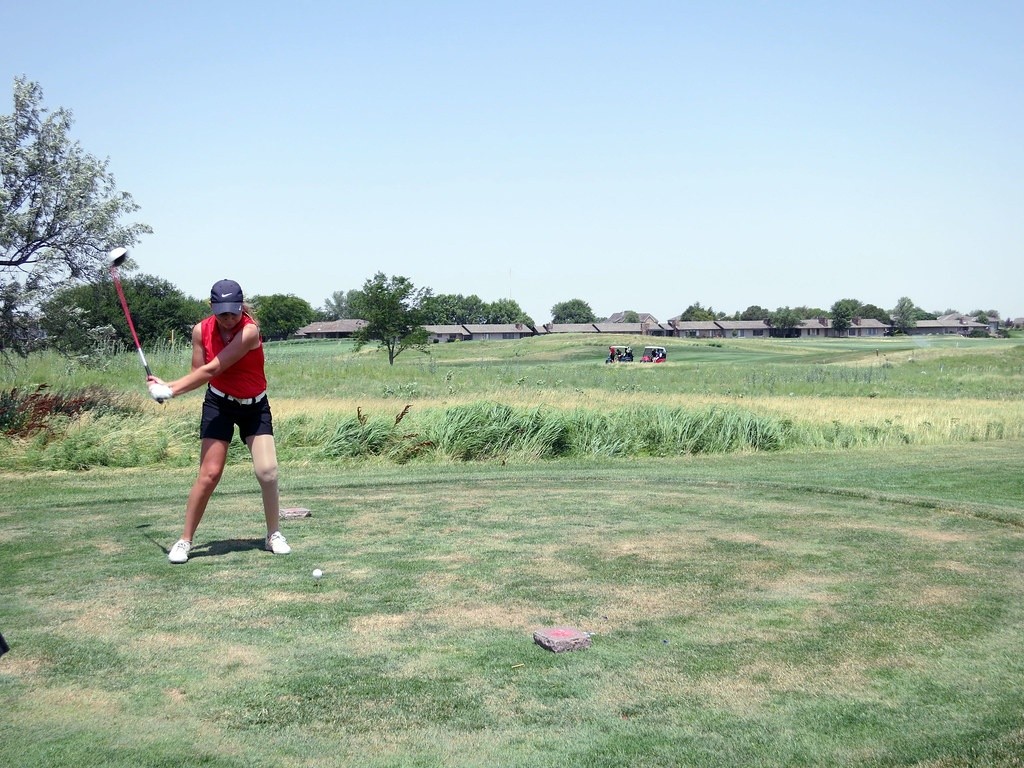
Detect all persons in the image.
[606,345,666,364]
[146,278,292,568]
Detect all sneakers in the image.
[168,538,193,563]
[265,530,290,555]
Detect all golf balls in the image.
[313,569,323,578]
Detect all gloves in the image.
[148,385,174,400]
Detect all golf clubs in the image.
[108,247,164,404]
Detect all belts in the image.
[209,385,266,403]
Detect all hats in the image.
[211,279,243,316]
